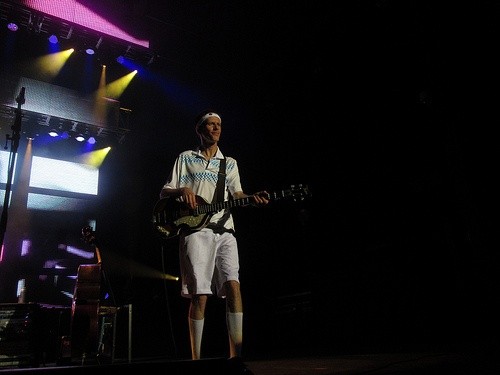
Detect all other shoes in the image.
[231,357,253,375]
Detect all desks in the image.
[38,303,120,364]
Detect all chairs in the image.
[0,304,40,368]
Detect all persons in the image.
[160,111,269,375]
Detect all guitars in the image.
[150,183,311,240]
